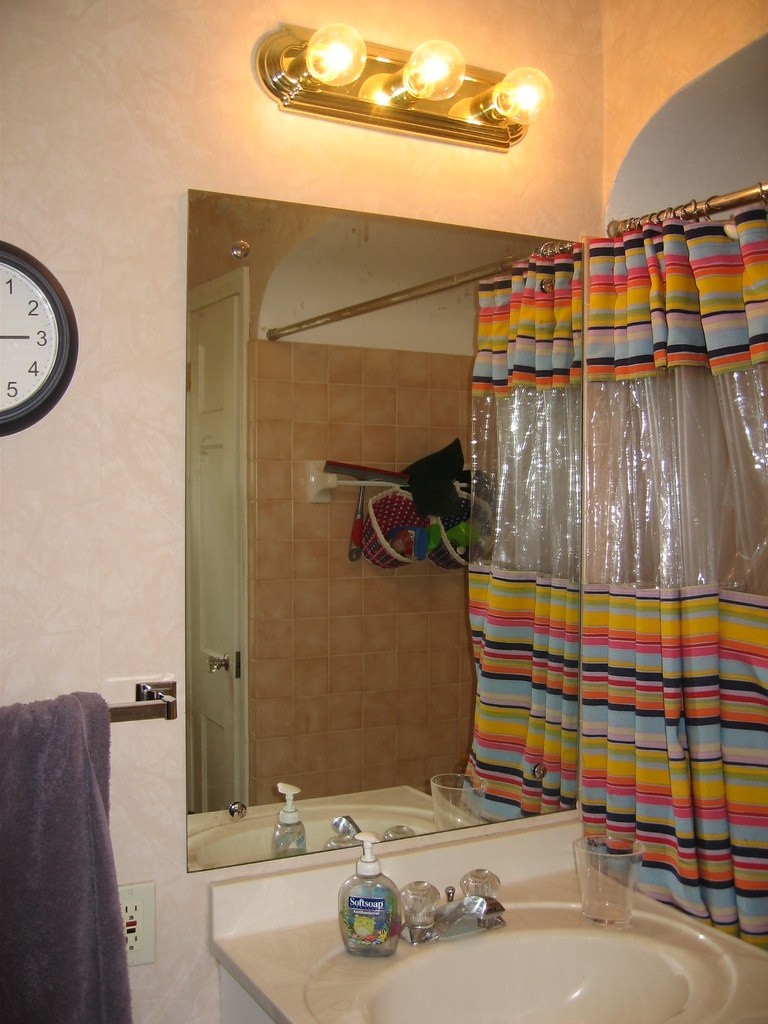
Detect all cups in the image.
[431,773,490,831]
[572,835,646,927]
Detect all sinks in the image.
[187,784,481,871]
[302,897,738,1024]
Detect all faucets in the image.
[330,812,361,837]
[433,892,507,933]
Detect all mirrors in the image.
[181,192,579,876]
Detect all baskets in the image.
[428,487,494,570]
[360,486,436,568]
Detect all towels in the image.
[0,685,134,1024]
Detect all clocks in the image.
[0,243,79,439]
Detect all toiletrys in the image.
[274,780,308,857]
[338,828,403,958]
